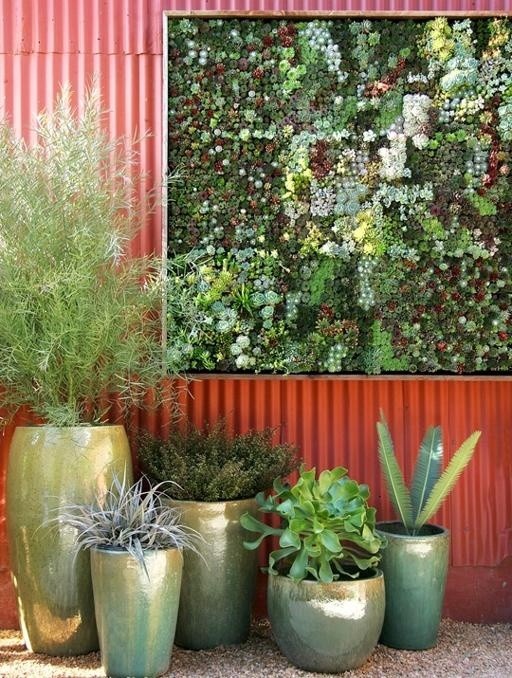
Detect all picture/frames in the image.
[158,7,511,385]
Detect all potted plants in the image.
[1,80,203,666]
[131,407,309,654]
[368,402,483,654]
[240,461,387,673]
[26,458,218,677]
